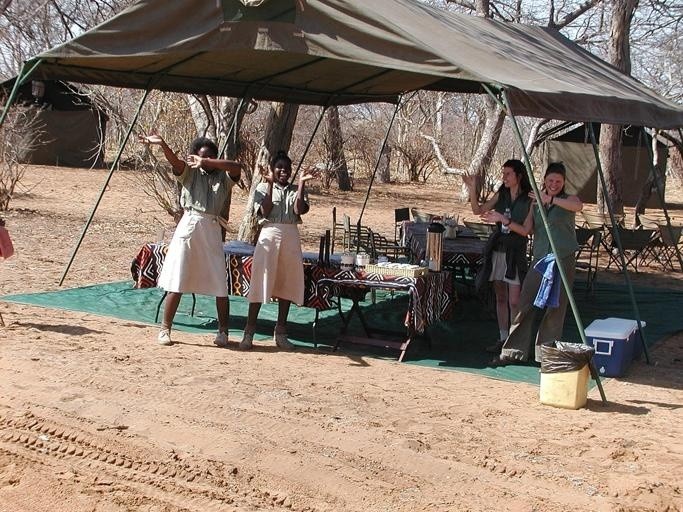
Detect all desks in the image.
[400,222,488,281]
[130,240,456,362]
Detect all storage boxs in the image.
[607,317,646,361]
[585,319,637,377]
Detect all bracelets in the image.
[550,194,553,204]
[503,217,511,227]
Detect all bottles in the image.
[501,207,512,234]
[424,222,445,274]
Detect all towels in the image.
[534,254,561,308]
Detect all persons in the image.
[475,159,587,369]
[135,123,241,348]
[460,155,537,345]
[236,150,316,354]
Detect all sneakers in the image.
[156,329,173,346]
[211,330,229,347]
[271,331,295,349]
[483,340,541,368]
[238,332,254,349]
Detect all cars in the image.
[316,162,354,177]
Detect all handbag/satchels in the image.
[236,210,262,247]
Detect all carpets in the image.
[0,274,683,395]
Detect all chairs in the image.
[331,208,414,264]
[575,211,683,291]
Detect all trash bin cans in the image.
[539,341,594,410]
